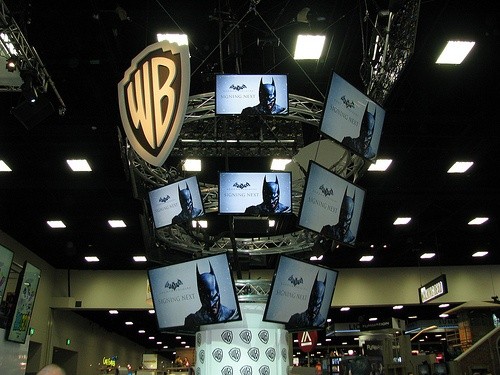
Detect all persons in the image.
[241,77,286,115]
[37,363,66,375]
[288,271,328,327]
[342,101,377,159]
[246,174,290,214]
[172,182,204,222]
[320,183,357,246]
[184,259,237,326]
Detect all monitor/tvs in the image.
[263,254,340,328]
[147,176,206,229]
[214,73,290,116]
[147,251,243,329]
[297,159,366,248]
[319,68,386,164]
[215,169,293,215]
